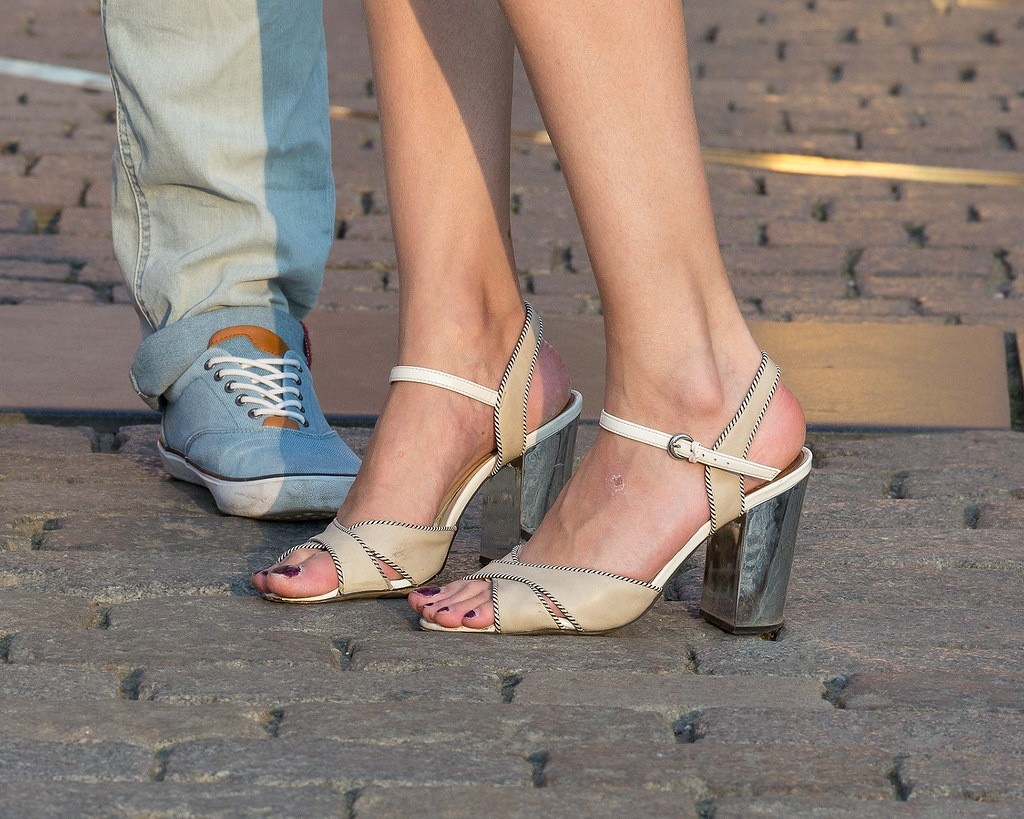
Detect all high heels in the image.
[263,298,584,605]
[419,349,814,637]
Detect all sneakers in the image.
[156,325,363,522]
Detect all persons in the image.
[104,0,366,521]
[252,0,813,635]
